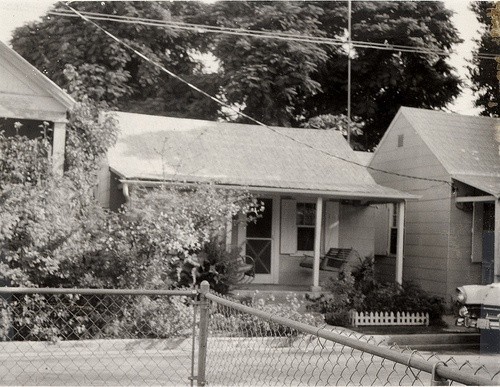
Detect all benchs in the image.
[299,248,354,273]
[235,254,255,287]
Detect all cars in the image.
[455,280,500,347]
